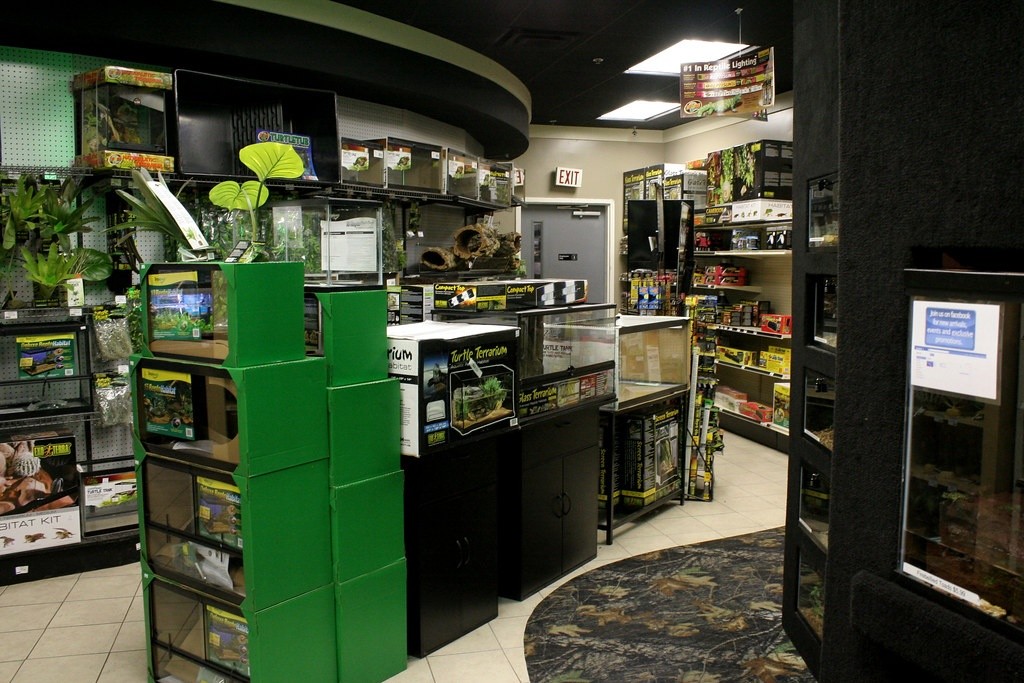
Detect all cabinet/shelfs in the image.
[690,207,792,455]
[519,408,604,600]
[403,442,497,658]
[0,168,509,594]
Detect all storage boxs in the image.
[134,227,791,683]
[618,140,793,227]
[73,65,175,172]
[15,332,76,380]
[341,135,515,208]
